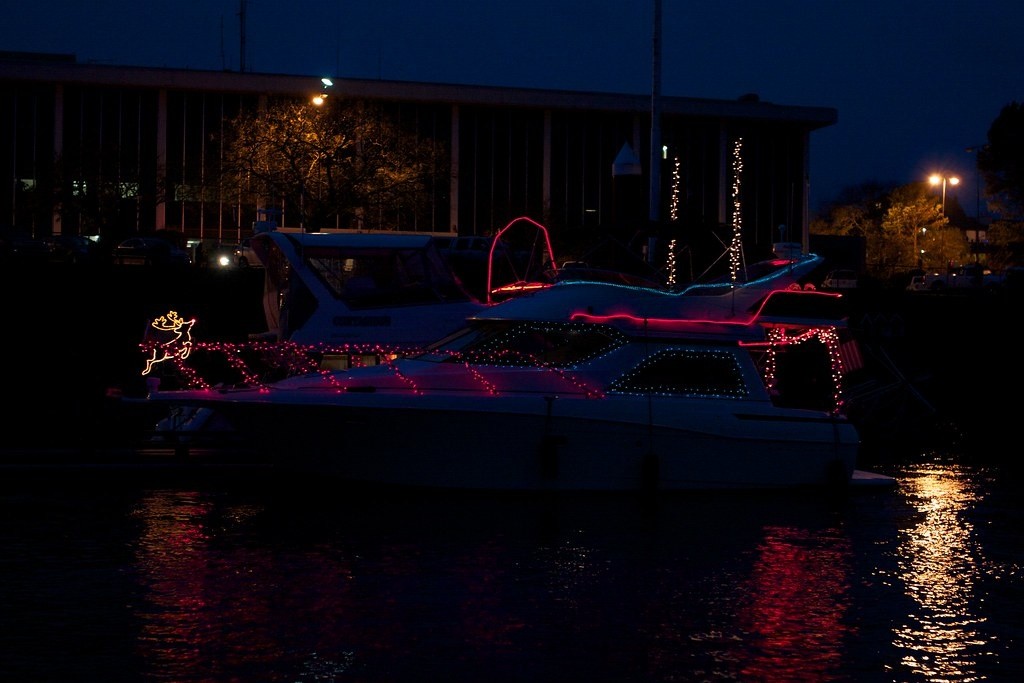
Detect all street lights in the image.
[964,143,992,263]
[926,169,963,274]
[311,94,325,203]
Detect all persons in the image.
[195,243,203,266]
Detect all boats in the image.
[109,229,871,487]
